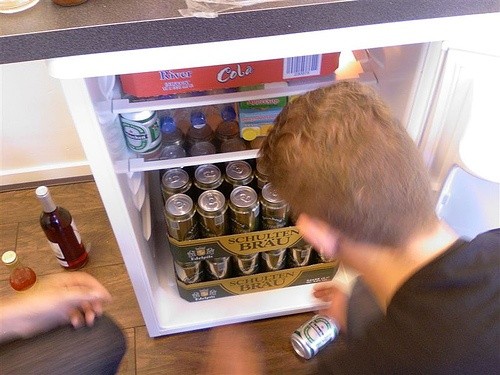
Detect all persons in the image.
[195,79,500,374]
[0,268,128,375]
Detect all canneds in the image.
[207,258,229,281]
[216,120,241,137]
[186,124,216,142]
[162,168,192,200]
[197,188,227,236]
[221,139,246,153]
[231,186,258,231]
[291,245,314,267]
[196,167,224,190]
[256,164,268,186]
[262,182,289,231]
[158,146,186,159]
[234,255,260,274]
[190,142,215,155]
[264,250,285,271]
[291,313,341,358]
[176,261,202,285]
[158,126,184,144]
[163,195,198,240]
[122,113,162,156]
[225,160,253,186]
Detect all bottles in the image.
[182,106,212,128]
[34,185,90,273]
[1,250,38,297]
[159,108,180,134]
[213,103,240,121]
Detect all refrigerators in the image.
[38,12,500,338]
